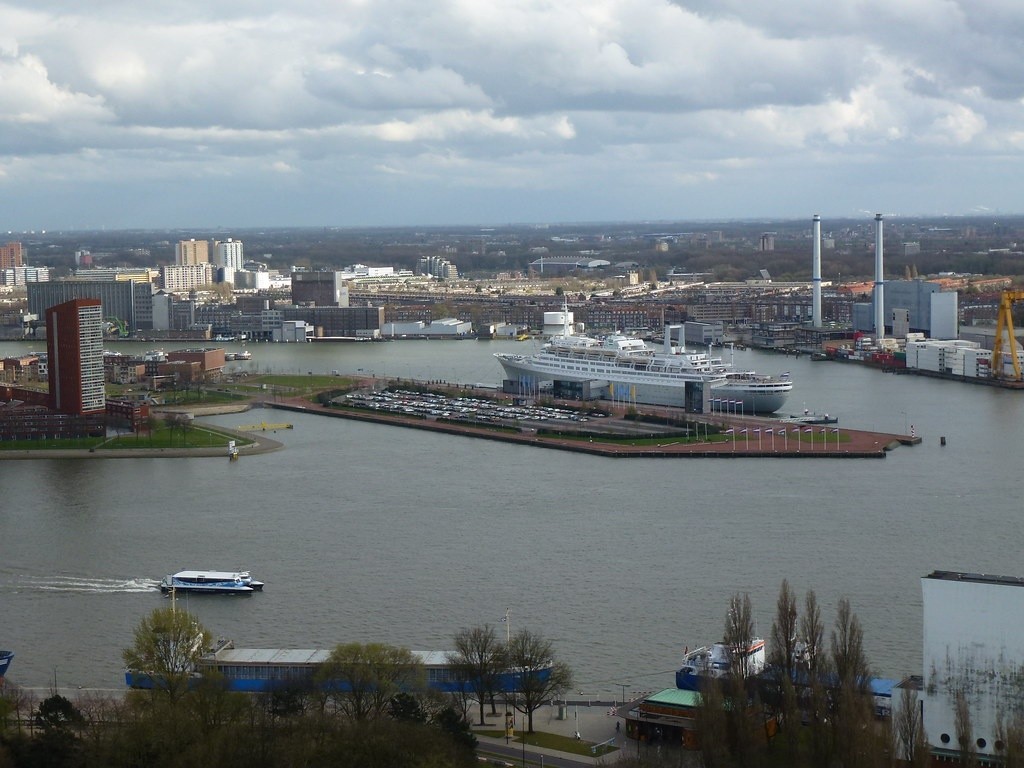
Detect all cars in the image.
[345,389,605,421]
[474,383,483,388]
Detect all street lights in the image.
[616,683,630,706]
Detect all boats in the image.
[125,621,552,694]
[159,570,265,595]
[0,650,14,677]
[789,409,838,423]
[493,303,793,413]
[675,633,901,719]
[225,350,251,361]
